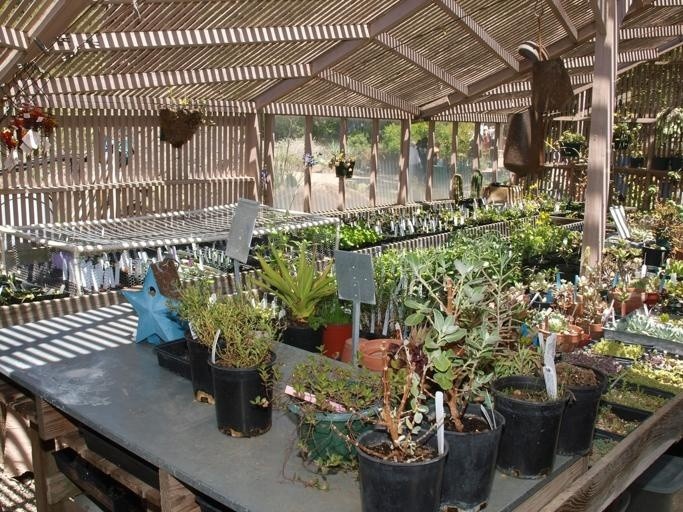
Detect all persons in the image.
[397,123,495,187]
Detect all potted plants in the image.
[326,152,355,178]
[612,105,683,171]
[558,120,583,157]
[408,243,521,510]
[206,290,283,439]
[250,228,681,479]
[282,346,386,491]
[359,257,489,511]
[175,263,241,405]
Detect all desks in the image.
[0,299,591,512]
